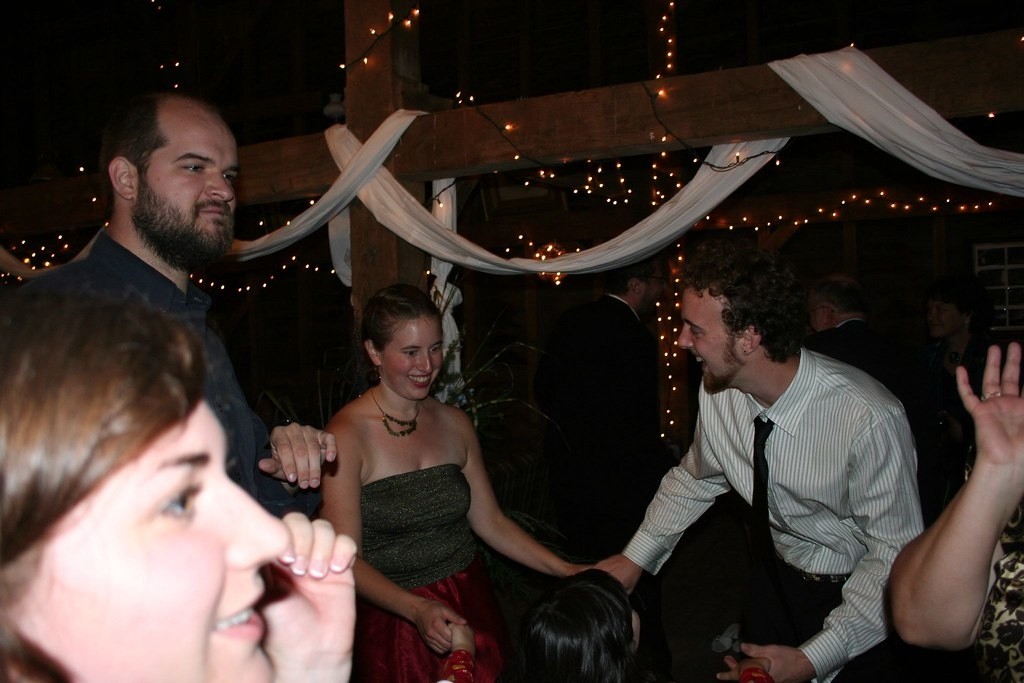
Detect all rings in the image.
[981,392,1001,400]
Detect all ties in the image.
[746,416,785,615]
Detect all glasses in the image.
[650,275,669,282]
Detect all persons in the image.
[12,84,336,522]
[0,284,358,682]
[318,282,596,683]
[797,266,1024,683]
[533,255,680,681]
[592,237,926,683]
[435,569,775,683]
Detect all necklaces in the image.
[371,388,419,436]
